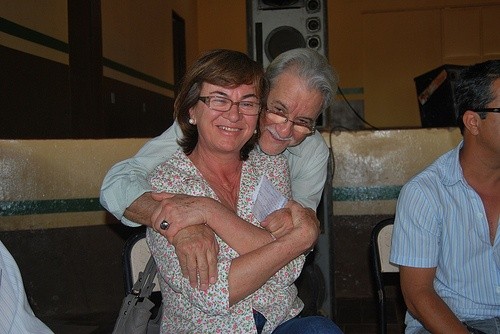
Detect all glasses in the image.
[198,94,263,116]
[264,106,316,136]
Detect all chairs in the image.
[121,147,333,320]
[370,217,400,334]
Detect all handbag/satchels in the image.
[112,255,161,334]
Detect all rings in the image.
[160,220,170,230]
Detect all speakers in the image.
[414,64,470,127]
[246,0,330,127]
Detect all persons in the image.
[145,48,346,334]
[390,61,500,334]
[100,48,339,290]
[0,241,54,334]
